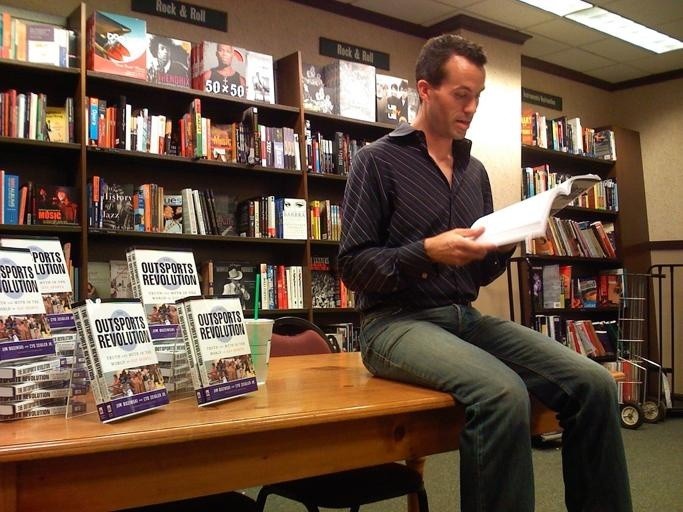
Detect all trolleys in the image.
[612,267,665,430]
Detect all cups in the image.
[243,319,276,385]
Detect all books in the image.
[521,110,639,407]
[0,11,74,143]
[303,59,418,125]
[1,170,80,225]
[462,172,600,255]
[87,10,275,104]
[0,238,258,423]
[304,120,374,242]
[85,95,309,240]
[259,255,361,354]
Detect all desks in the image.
[0,352,624,512]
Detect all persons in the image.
[334,36,632,511]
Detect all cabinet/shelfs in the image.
[0,0,400,354]
[521,126,661,402]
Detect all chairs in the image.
[116,491,256,512]
[256,316,429,512]
